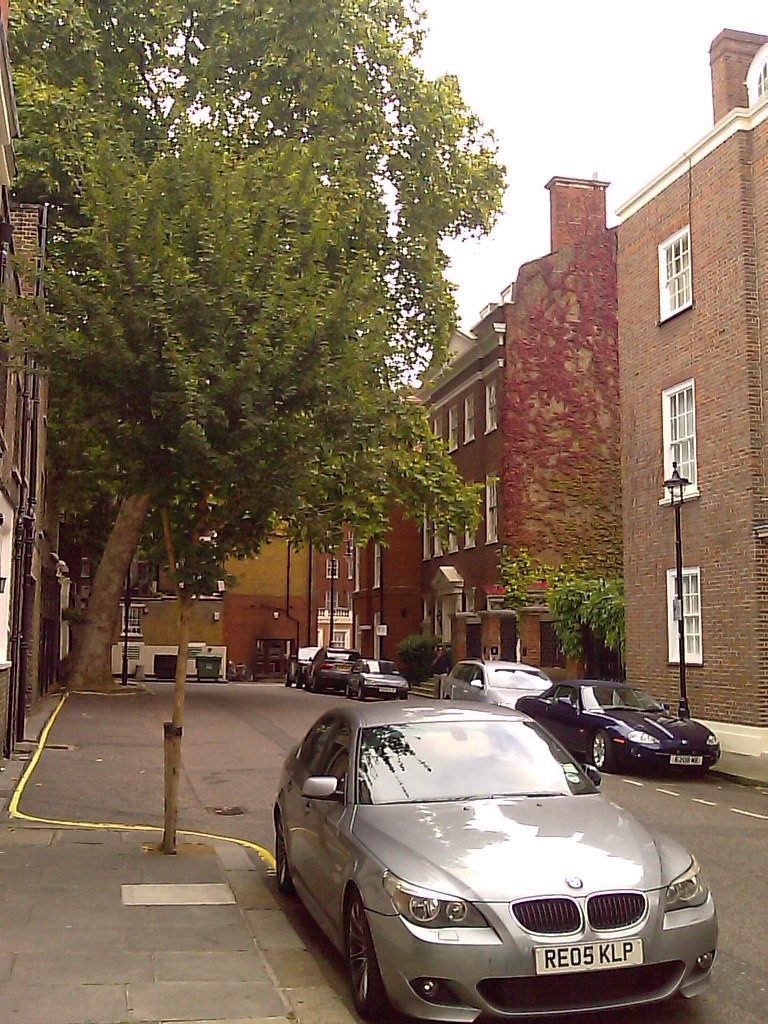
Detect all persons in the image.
[431,644,449,700]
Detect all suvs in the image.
[304,646,361,694]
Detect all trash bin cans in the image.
[195,656,223,683]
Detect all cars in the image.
[285,646,322,689]
[514,677,721,779]
[441,657,553,712]
[346,658,410,700]
[272,699,717,1024]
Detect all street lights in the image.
[663,461,692,721]
